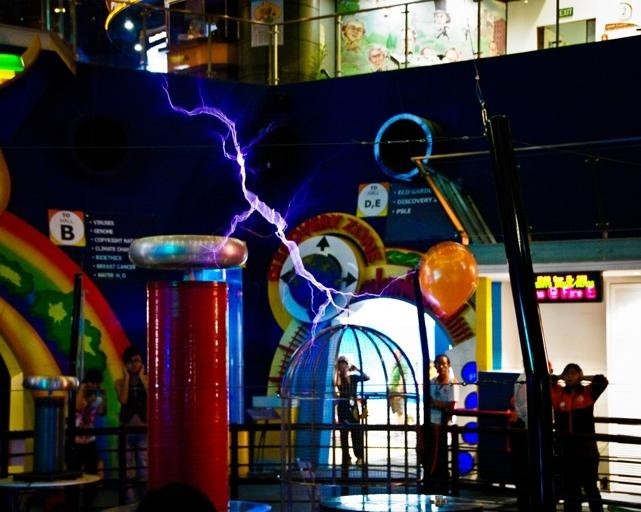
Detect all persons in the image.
[421,351,461,492]
[547,361,611,511]
[331,355,370,467]
[67,366,108,476]
[112,344,150,499]
[384,348,416,425]
